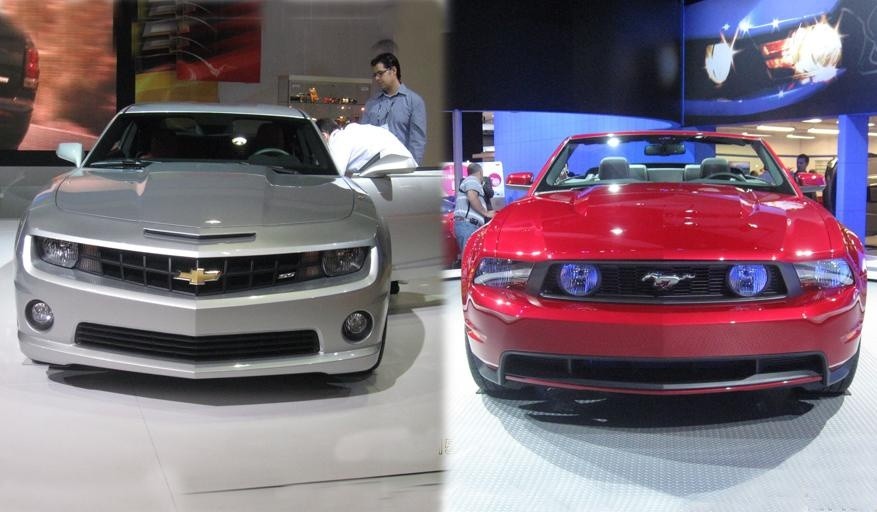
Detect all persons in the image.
[314,117,420,176]
[452,163,496,254]
[458,175,494,223]
[356,52,427,167]
[790,153,816,202]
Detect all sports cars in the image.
[456,128,868,411]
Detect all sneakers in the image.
[452,259,461,269]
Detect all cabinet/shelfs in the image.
[277,74,374,126]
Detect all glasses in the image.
[372,69,388,77]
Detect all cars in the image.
[10,99,400,390]
[438,192,498,275]
[0,15,42,154]
[682,1,848,125]
